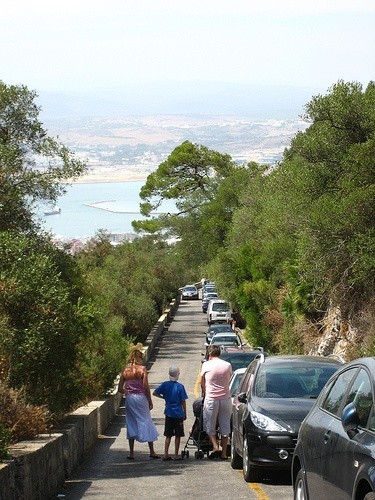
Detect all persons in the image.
[152,366,188,460]
[201,345,232,460]
[118,349,160,459]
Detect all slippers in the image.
[174,457,184,460]
[220,455,228,460]
[211,450,221,458]
[149,455,159,458]
[162,457,173,461]
[127,455,135,459]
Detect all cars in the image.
[201,323,269,419]
[181,282,219,313]
[291,356,375,500]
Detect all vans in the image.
[206,299,233,325]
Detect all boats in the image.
[42,207,61,215]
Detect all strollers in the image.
[182,397,220,460]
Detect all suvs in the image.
[229,354,347,485]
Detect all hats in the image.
[169,366,179,380]
